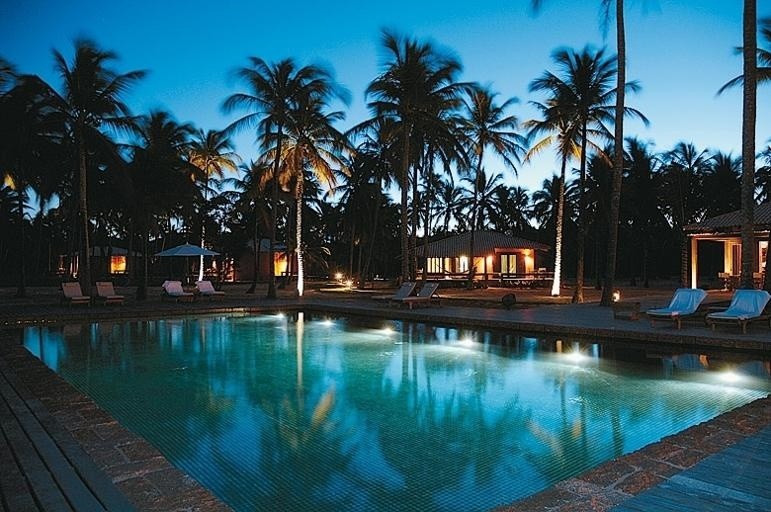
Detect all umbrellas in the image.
[156,241,220,292]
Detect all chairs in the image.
[160,278,225,304]
[372,280,444,308]
[647,288,771,335]
[59,280,124,310]
